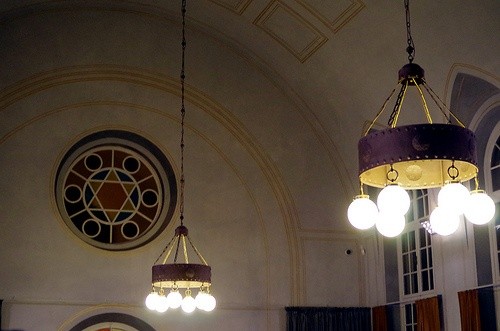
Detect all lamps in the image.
[146,1,216,314]
[346,0,497,238]
